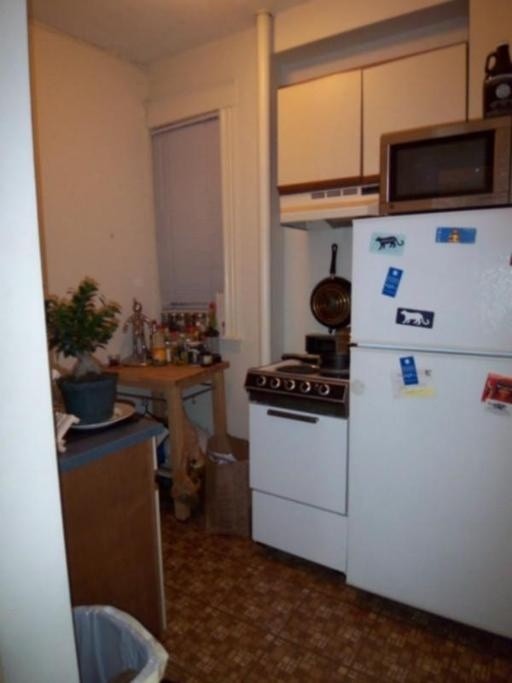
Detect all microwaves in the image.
[376,113,511,211]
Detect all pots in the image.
[282,350,349,376]
[310,241,351,329]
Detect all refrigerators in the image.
[343,210,511,646]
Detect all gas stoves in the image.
[243,359,348,401]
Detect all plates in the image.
[67,401,136,430]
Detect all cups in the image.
[108,352,120,366]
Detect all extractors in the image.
[278,182,379,232]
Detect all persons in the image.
[123,296,156,363]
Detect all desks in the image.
[107,358,234,520]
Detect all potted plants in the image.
[43,274,128,425]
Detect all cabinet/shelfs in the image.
[59,415,173,639]
[276,37,470,193]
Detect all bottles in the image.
[150,309,221,366]
[483,41,512,116]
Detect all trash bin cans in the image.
[72,605,169,683]
